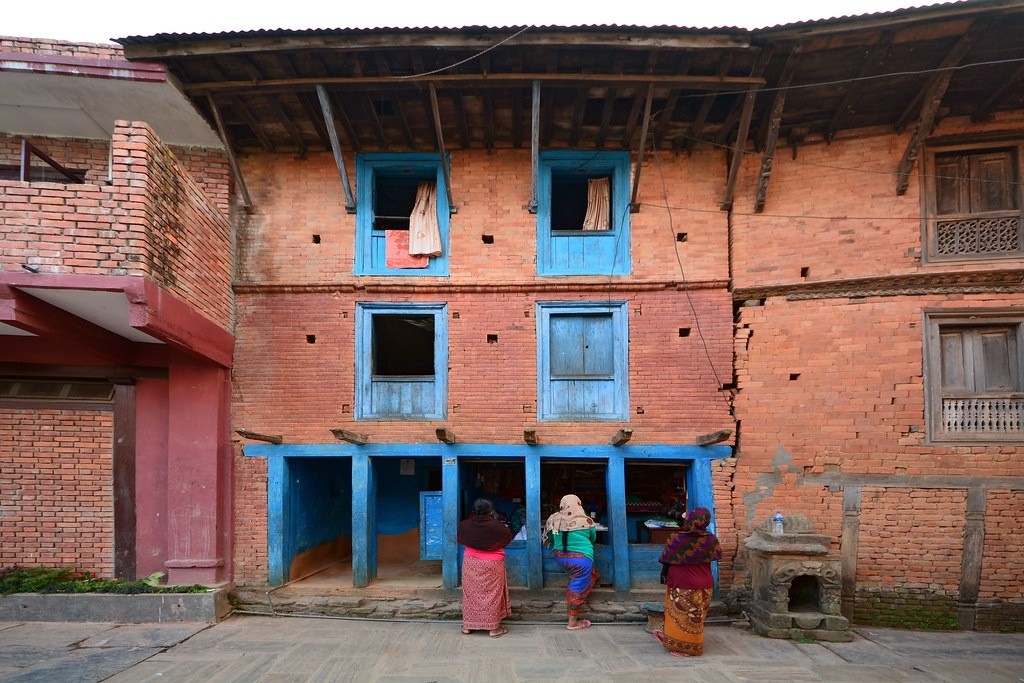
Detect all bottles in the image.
[772,511,784,534]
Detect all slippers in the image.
[461,624,471,635]
[488,628,509,638]
[579,603,591,612]
[652,628,665,646]
[568,619,591,630]
[670,650,690,657]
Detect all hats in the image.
[473,499,494,514]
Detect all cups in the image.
[591,512,596,518]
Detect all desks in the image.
[636,521,682,544]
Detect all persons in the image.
[541,494,596,630]
[456,500,512,639]
[653,508,722,657]
